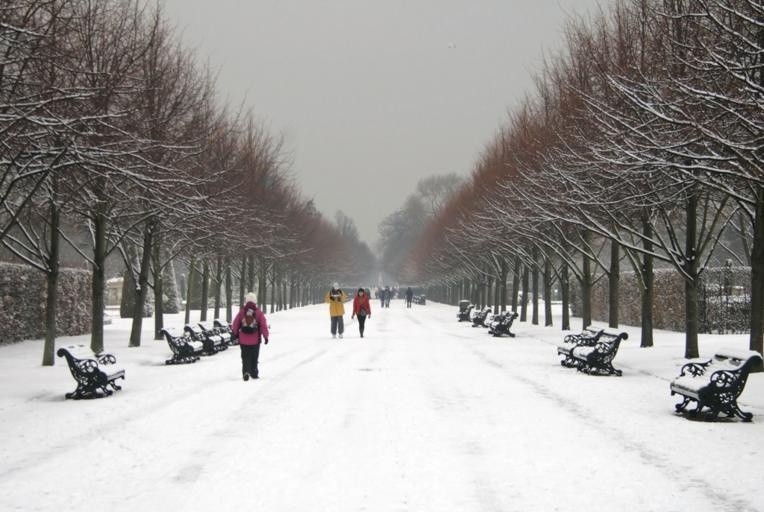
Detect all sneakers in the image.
[244,374,258,381]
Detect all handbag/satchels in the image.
[361,308,367,317]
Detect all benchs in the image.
[456,305,518,336]
[158,319,240,364]
[669,347,763,423]
[572,327,628,376]
[57,343,125,399]
[557,325,605,368]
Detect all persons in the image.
[231,292,269,381]
[325,282,347,340]
[351,287,371,338]
[375,285,413,309]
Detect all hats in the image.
[246,292,258,305]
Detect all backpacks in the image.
[239,308,259,334]
